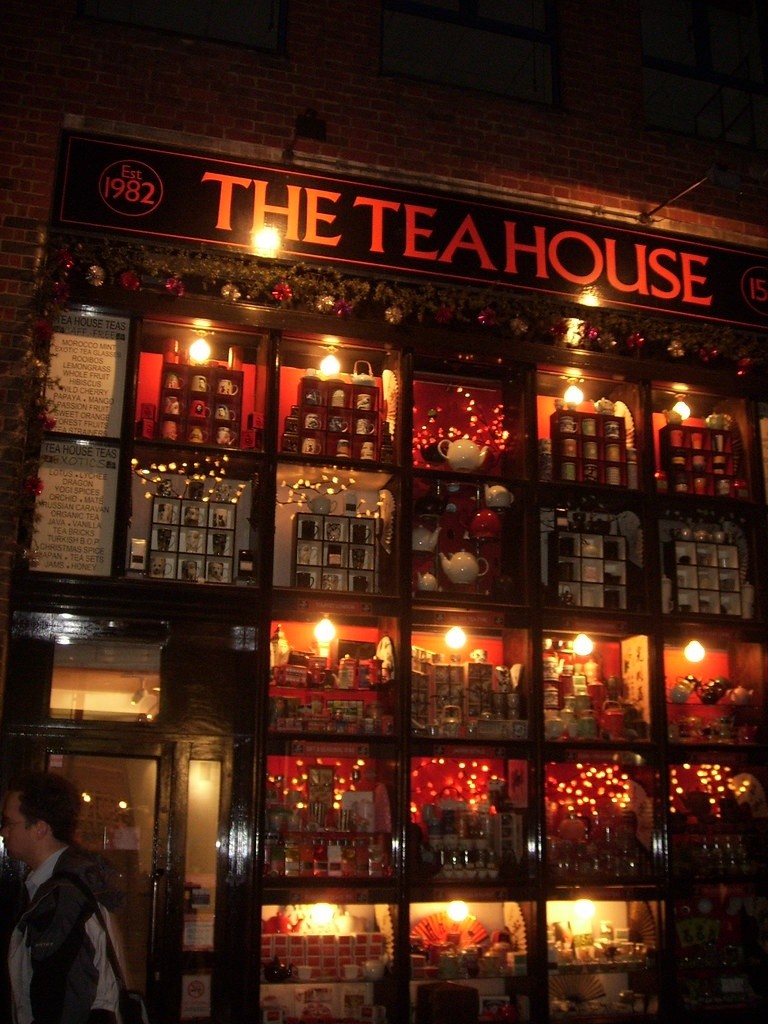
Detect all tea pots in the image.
[593,396,615,416]
[540,652,647,741]
[409,924,518,981]
[437,439,489,473]
[412,485,514,591]
[569,512,612,535]
[705,412,725,430]
[264,956,293,982]
[666,672,759,744]
[305,493,337,515]
[271,624,395,735]
[663,410,683,426]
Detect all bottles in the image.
[545,804,644,886]
[671,834,752,877]
[297,520,372,592]
[405,796,508,881]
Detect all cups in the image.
[657,426,749,500]
[151,501,231,583]
[538,409,626,486]
[298,965,313,979]
[410,655,528,744]
[299,387,376,461]
[342,965,360,979]
[546,531,625,608]
[344,494,358,517]
[660,540,743,616]
[154,364,240,447]
[670,520,735,544]
[553,398,576,411]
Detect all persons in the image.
[0,770,124,1024]
